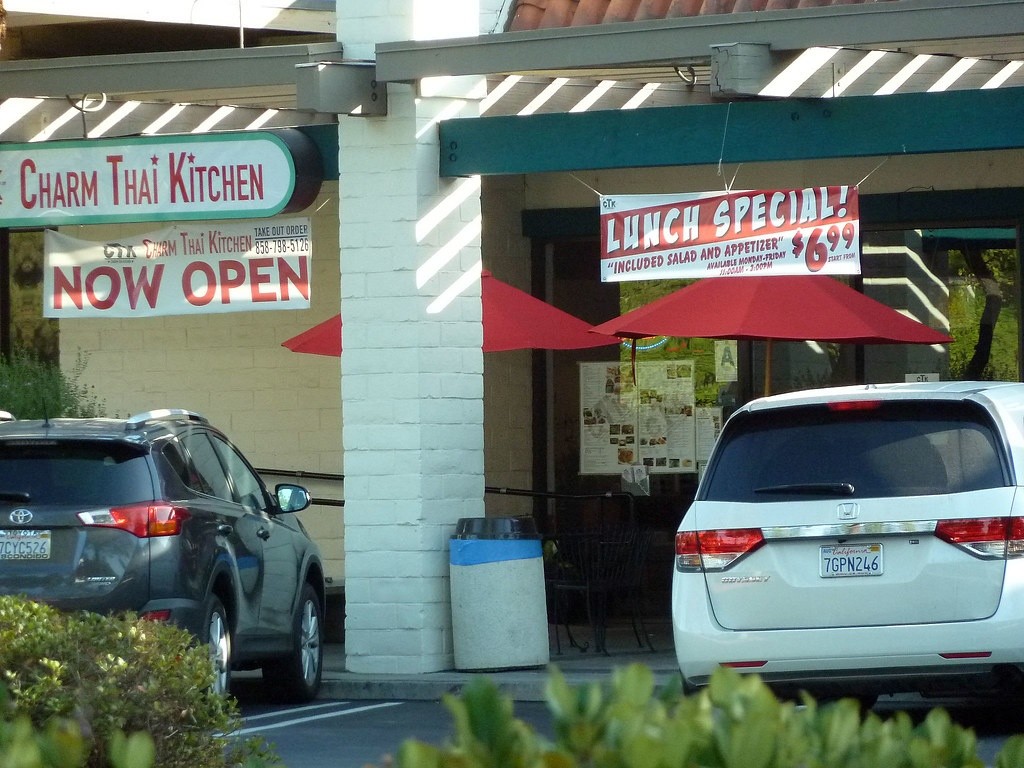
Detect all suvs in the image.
[0,408,325,714]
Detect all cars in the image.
[670,380,1024,730]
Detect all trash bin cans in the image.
[448,515,551,672]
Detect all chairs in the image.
[544,522,656,657]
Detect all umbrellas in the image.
[587,275,955,399]
[281,268,624,356]
